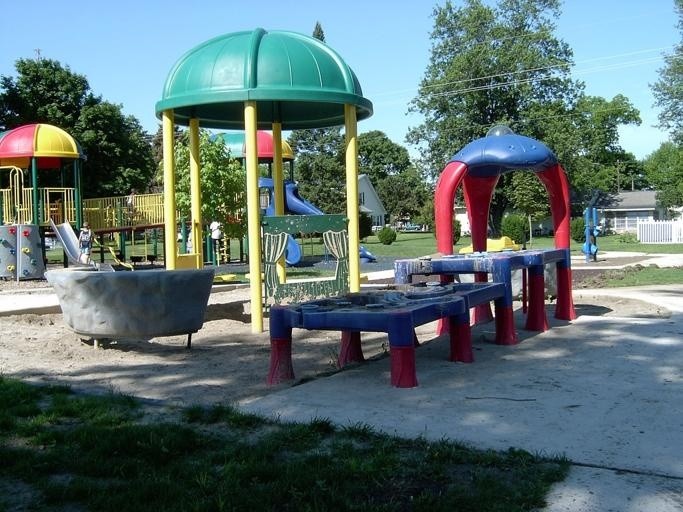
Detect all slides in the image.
[258,177,376,266]
[49,217,97,267]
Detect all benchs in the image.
[44,267,215,349]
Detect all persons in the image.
[76,221,90,265]
[87,225,98,264]
[177,219,223,253]
[127,188,140,218]
